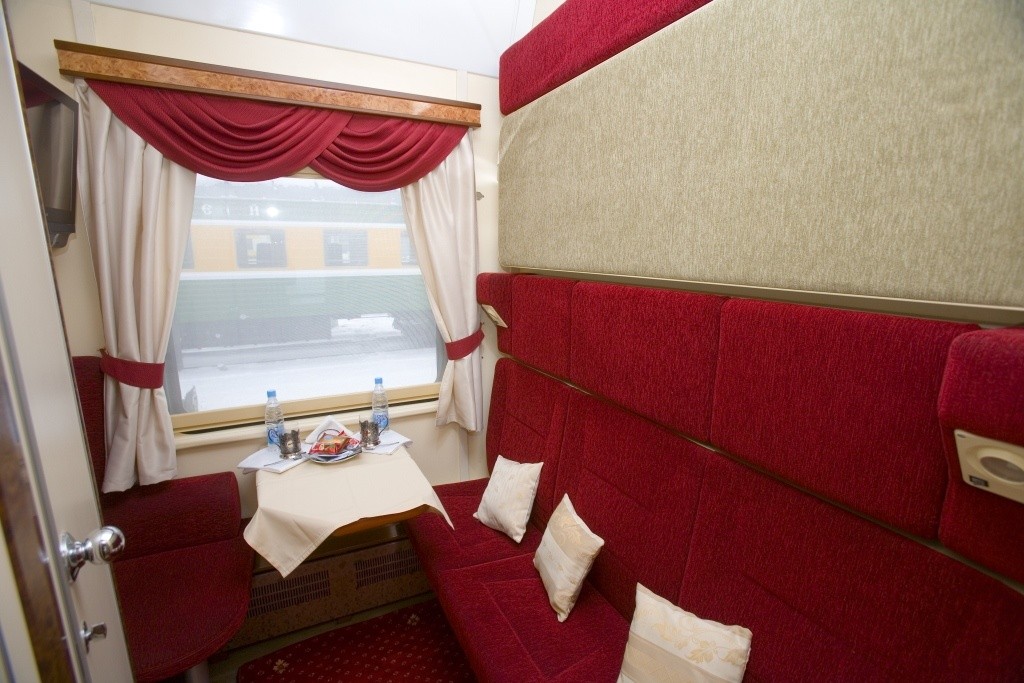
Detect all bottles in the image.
[264,390,285,452]
[372,378,388,432]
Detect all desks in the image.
[250,436,433,541]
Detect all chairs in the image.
[67,354,257,681]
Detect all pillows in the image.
[618,579,754,683]
[531,494,604,623]
[470,449,546,545]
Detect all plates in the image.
[306,450,361,464]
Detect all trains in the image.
[170,169,452,352]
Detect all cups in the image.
[357,412,387,449]
[268,421,302,460]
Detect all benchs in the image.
[401,265,1024,683]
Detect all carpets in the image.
[232,601,469,683]
